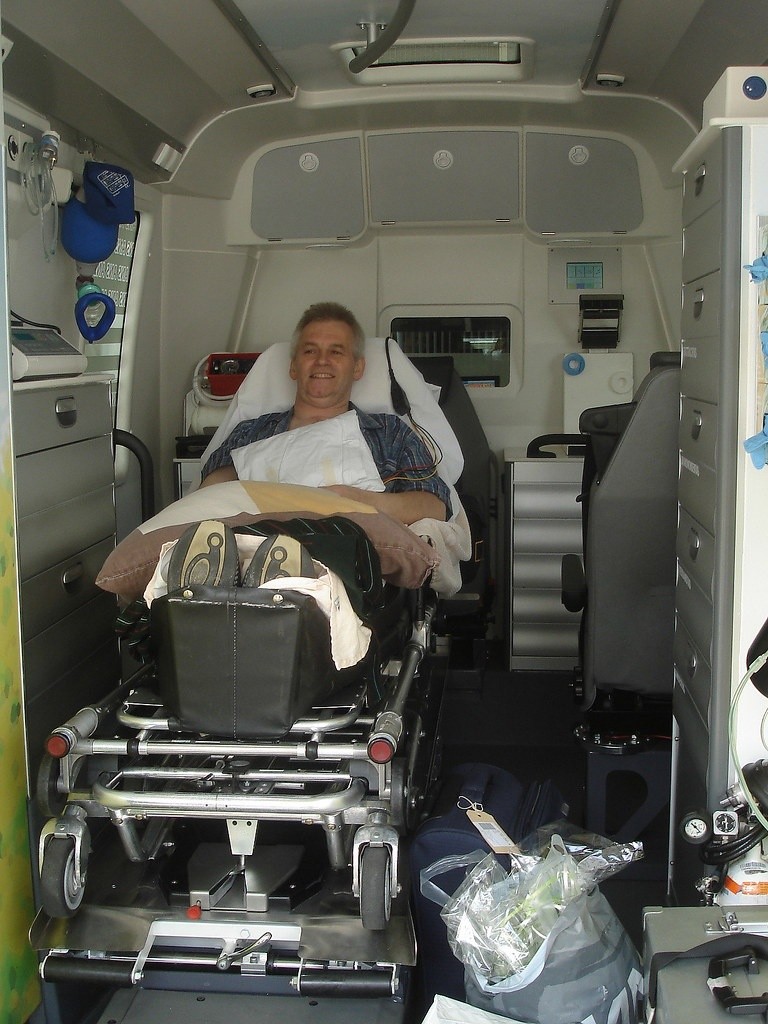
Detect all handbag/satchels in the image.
[418,848,646,1024]
[408,762,585,1013]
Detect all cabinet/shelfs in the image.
[10,373,122,799]
[671,118,768,907]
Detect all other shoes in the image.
[167,520,240,594]
[242,533,314,588]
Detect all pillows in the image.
[230,409,386,494]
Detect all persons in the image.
[196,302,454,590]
